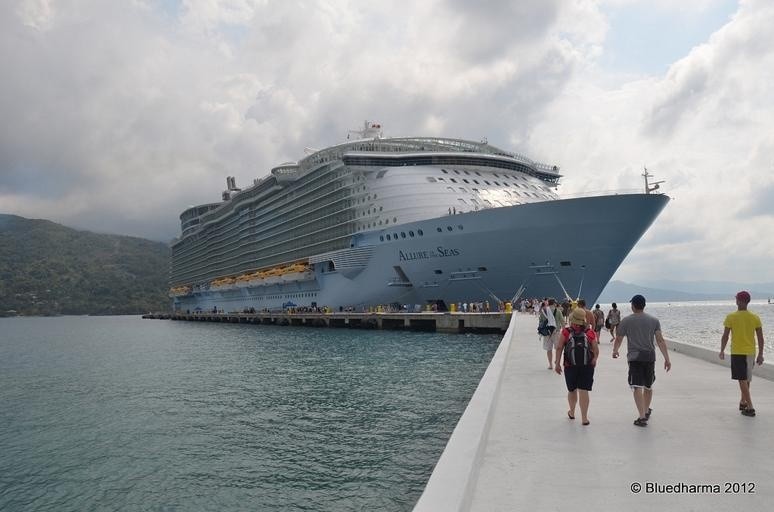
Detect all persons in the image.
[555,308,599,425]
[213,300,490,314]
[448,208,451,216]
[500,295,621,369]
[720,292,764,417]
[613,295,671,427]
[453,207,455,214]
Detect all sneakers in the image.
[740,402,746,410]
[742,409,755,416]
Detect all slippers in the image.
[568,411,575,419]
[582,421,589,425]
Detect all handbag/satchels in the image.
[538,326,555,335]
[605,319,611,329]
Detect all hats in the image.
[569,308,587,325]
[737,291,750,302]
[630,295,645,306]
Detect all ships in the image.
[168,119,672,313]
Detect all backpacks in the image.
[564,326,591,366]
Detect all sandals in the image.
[645,408,651,419]
[634,418,648,426]
